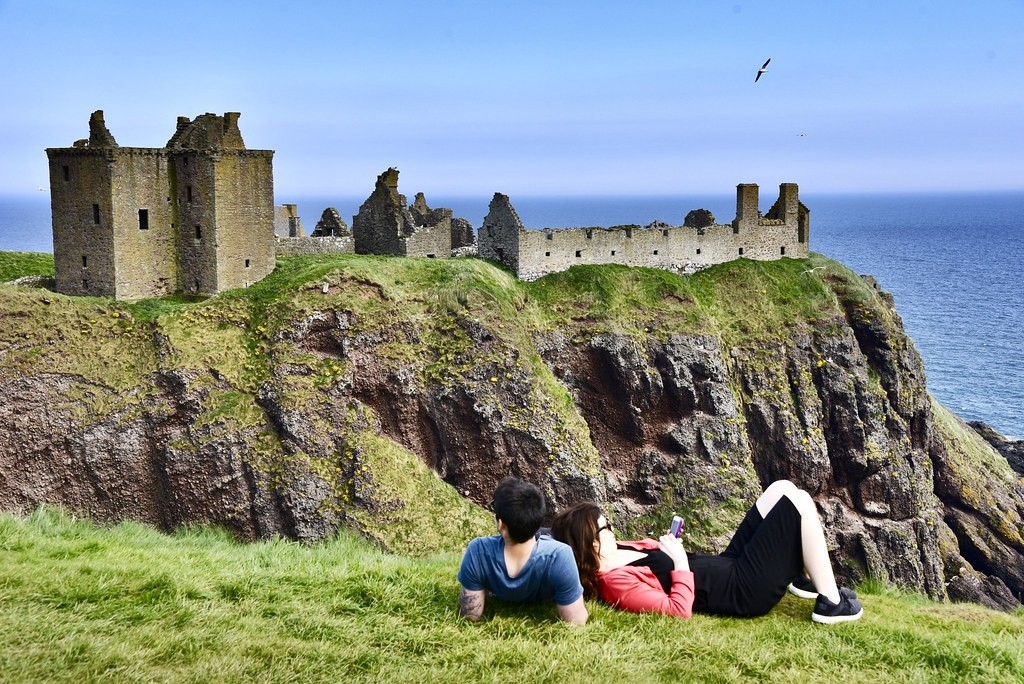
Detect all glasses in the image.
[595,518,612,534]
[487,500,502,520]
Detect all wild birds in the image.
[754,57,772,83]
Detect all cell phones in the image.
[670,515,683,539]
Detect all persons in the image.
[457,477,589,625]
[551,479,863,624]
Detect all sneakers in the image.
[789,575,856,599]
[812,585,863,623]
